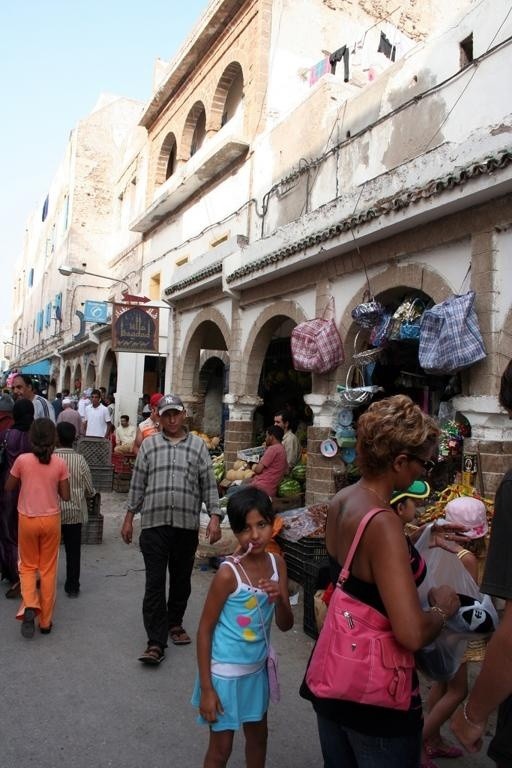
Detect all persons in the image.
[82,387,115,439]
[190,488,294,768]
[300,360,511,767]
[274,410,300,469]
[121,395,221,666]
[113,415,138,454]
[136,393,163,444]
[221,424,286,498]
[2,368,95,639]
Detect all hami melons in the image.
[227,461,258,481]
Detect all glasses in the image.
[404,452,436,472]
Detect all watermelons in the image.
[276,477,300,497]
[290,465,306,481]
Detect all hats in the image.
[443,497,488,539]
[389,480,431,504]
[150,393,164,407]
[157,395,183,416]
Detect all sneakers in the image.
[21,607,35,639]
[5,583,20,598]
[39,621,52,634]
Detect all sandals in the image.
[424,743,463,759]
[420,755,439,767]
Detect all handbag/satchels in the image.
[0,447,9,473]
[305,588,415,713]
[352,330,383,368]
[351,289,383,329]
[399,298,426,342]
[338,365,374,408]
[267,646,280,706]
[290,318,344,376]
[418,291,488,378]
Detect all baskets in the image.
[303,559,334,641]
[274,534,325,587]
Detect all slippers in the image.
[169,627,191,645]
[138,647,165,664]
